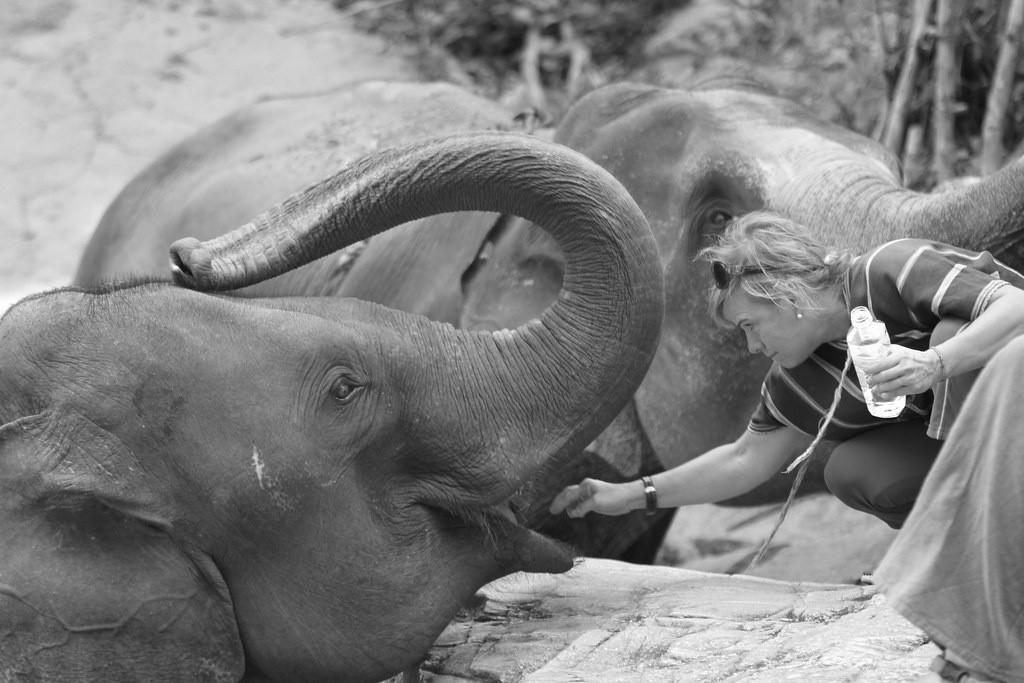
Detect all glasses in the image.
[711,260,777,289]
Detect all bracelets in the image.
[929,346,947,384]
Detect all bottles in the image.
[848,306,906,418]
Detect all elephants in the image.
[0,83,1024,683]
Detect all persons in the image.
[550,209,1024,682]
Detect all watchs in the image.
[640,476,659,518]
